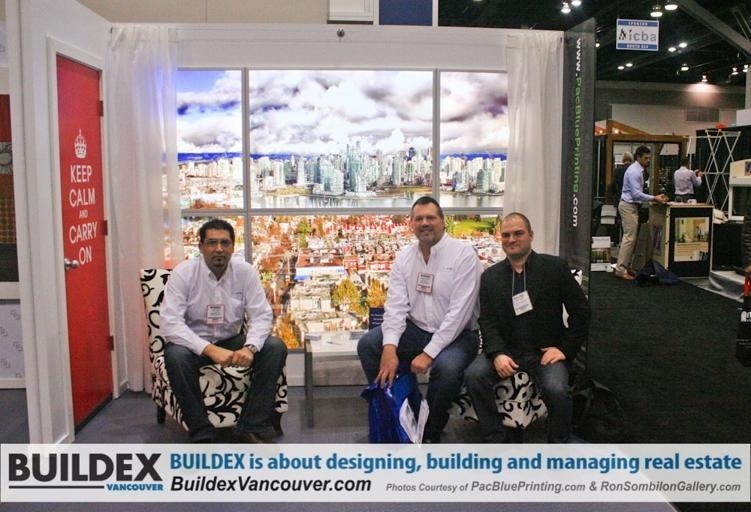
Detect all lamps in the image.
[698,60,751,82]
[650,0,679,18]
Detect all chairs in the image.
[137,268,288,436]
[423,268,583,444]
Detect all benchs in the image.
[303,331,373,427]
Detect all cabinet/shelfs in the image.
[647,201,715,280]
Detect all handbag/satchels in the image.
[636,259,680,287]
[361,361,430,443]
[571,378,629,443]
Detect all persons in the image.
[614,146,668,280]
[357,196,486,443]
[462,211,592,444]
[673,155,703,203]
[159,218,289,442]
[609,152,635,246]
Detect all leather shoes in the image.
[549,426,569,442]
[246,432,265,443]
[616,269,635,280]
